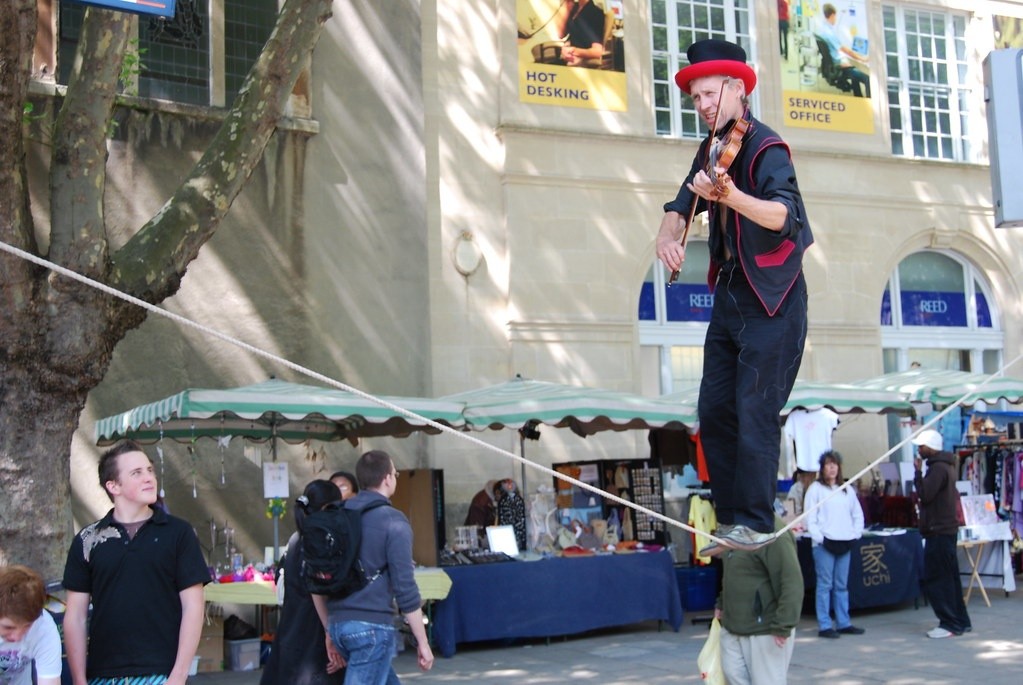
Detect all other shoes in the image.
[836,625,865,635]
[926,626,954,638]
[818,628,840,639]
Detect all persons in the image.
[655,38,815,558]
[0,430,972,685]
[536,0,871,99]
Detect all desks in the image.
[430,551,683,658]
[204,566,452,650]
[796,527,925,617]
[956,534,1016,607]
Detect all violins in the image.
[700,117,750,200]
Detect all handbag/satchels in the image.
[696,616,726,685]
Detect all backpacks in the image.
[299,497,393,600]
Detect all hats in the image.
[675,39,757,96]
[911,429,943,451]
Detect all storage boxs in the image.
[224,637,262,671]
[196,606,224,672]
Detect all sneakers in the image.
[720,523,777,551]
[699,523,736,557]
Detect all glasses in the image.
[385,472,400,480]
[339,485,349,491]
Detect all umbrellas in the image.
[92,363,1022,579]
